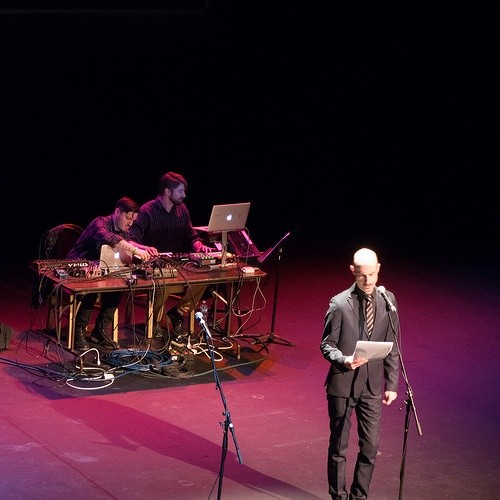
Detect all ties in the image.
[365,295,374,336]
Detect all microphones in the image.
[377,285,398,313]
[194,312,212,340]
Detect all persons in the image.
[65,198,149,351]
[124,172,211,338]
[320,248,400,500]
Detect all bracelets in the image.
[132,247,137,254]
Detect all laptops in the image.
[191,203,251,232]
[89,244,133,270]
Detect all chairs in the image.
[127,286,195,339]
[44,224,119,348]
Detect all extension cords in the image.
[79,371,114,382]
[171,348,198,356]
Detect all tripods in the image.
[248,247,296,346]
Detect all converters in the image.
[125,274,137,285]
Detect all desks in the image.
[34,262,267,374]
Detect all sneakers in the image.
[153,322,163,337]
[166,307,188,336]
[90,329,120,348]
[74,328,89,351]
